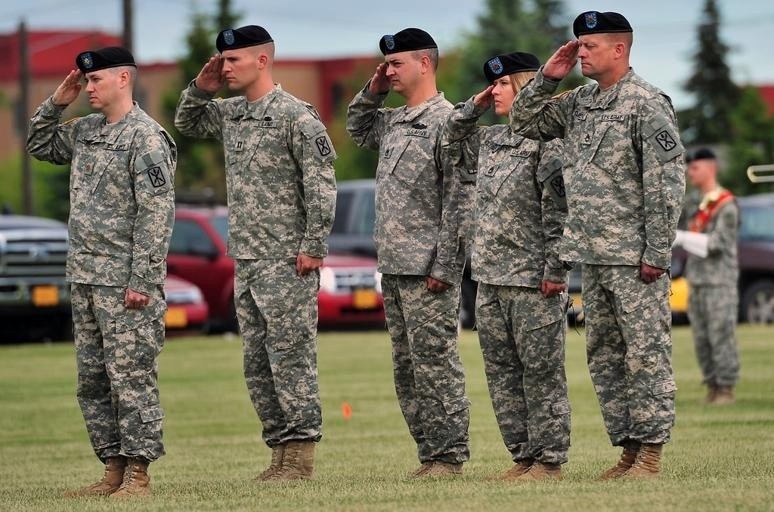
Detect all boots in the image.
[402,459,433,482]
[252,443,284,481]
[412,460,464,481]
[107,456,153,498]
[266,438,316,479]
[58,453,128,497]
[513,461,562,481]
[617,441,663,481]
[497,458,535,481]
[595,441,642,482]
[703,383,734,407]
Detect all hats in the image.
[76,45,137,77]
[215,22,275,55]
[483,51,542,85]
[684,147,716,163]
[572,12,636,39]
[378,27,438,57]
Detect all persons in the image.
[509,12,687,481]
[346,28,470,480]
[27,44,174,499]
[175,24,338,485]
[450,52,572,480]
[672,146,740,410]
